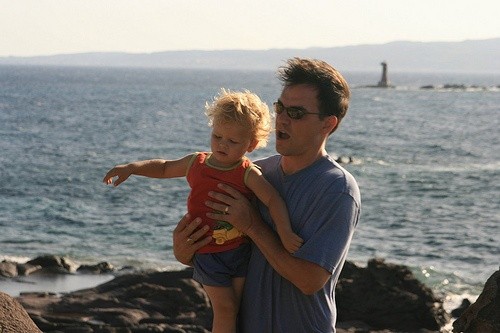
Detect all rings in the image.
[188,236,194,245]
[224,205,229,214]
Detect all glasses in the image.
[272,101,332,121]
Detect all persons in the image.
[172,59,363,333]
[102,93,304,332]
